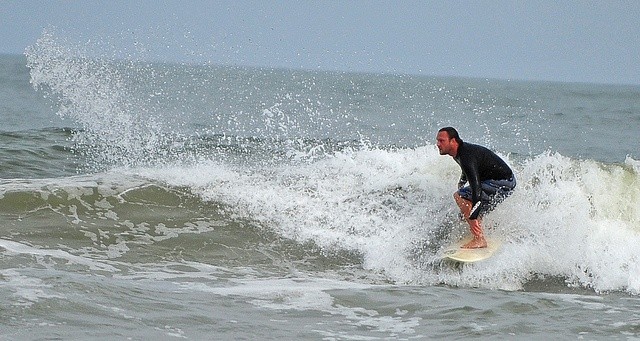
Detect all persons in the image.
[436,127,517,249]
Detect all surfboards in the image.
[444,232,503,263]
